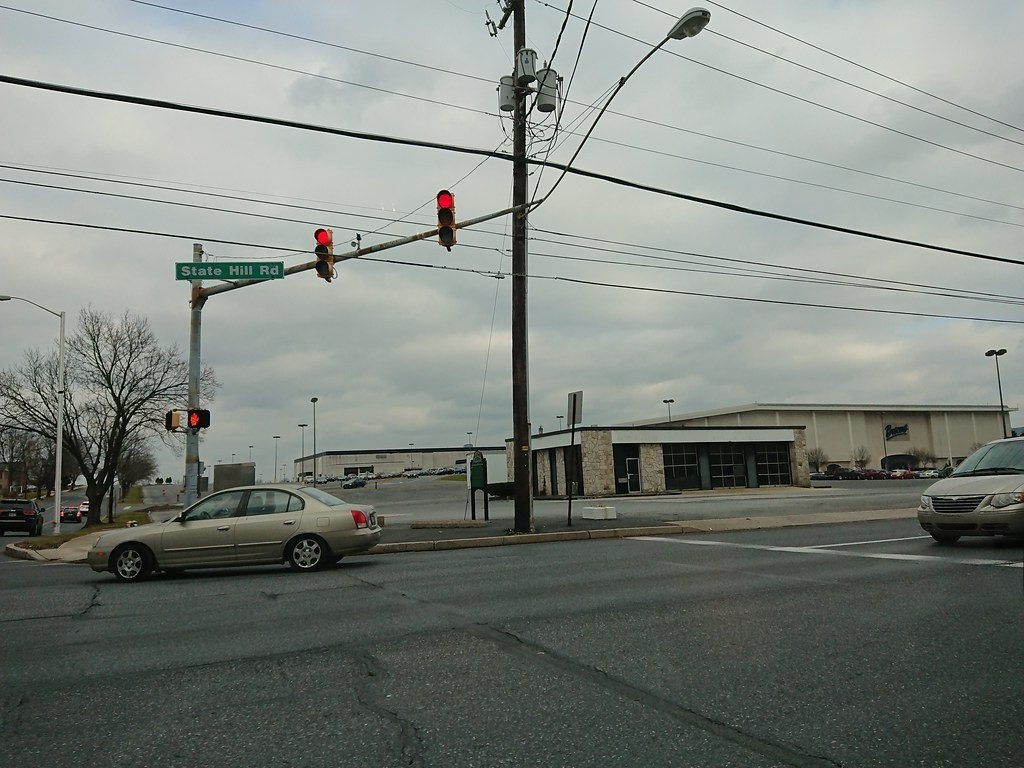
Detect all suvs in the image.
[1,498,46,538]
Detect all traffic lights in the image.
[166,410,179,430]
[188,410,211,428]
[315,227,334,280]
[436,190,457,247]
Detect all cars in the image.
[83,484,382,584]
[304,466,467,489]
[917,437,1023,545]
[80,501,90,515]
[60,506,82,523]
[809,464,957,480]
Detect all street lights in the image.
[0,294,69,535]
[556,416,564,432]
[467,432,473,454]
[283,463,286,483]
[507,5,713,535]
[249,445,254,464]
[409,444,414,469]
[984,348,1011,439]
[663,399,674,424]
[310,397,319,486]
[273,436,282,483]
[232,454,236,463]
[298,424,309,485]
